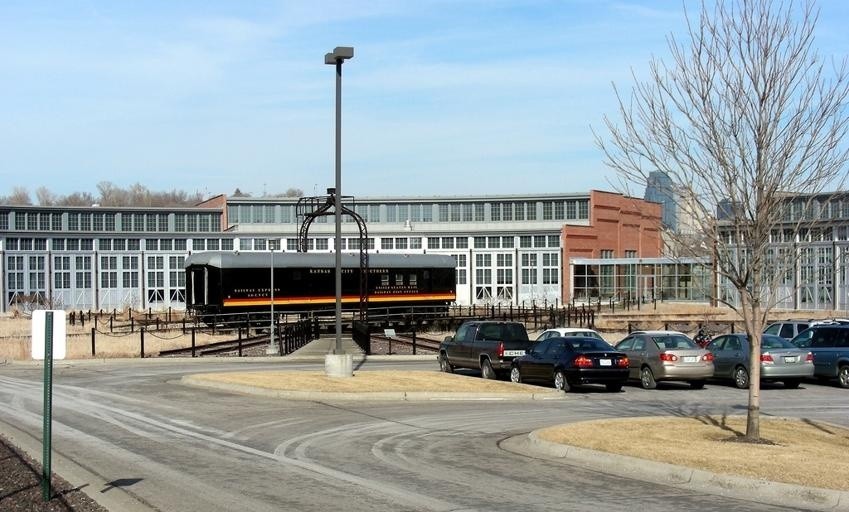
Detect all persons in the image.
[694,329,711,346]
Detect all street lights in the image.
[324,46,355,354]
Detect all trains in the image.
[183,252,457,333]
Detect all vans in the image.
[787,321,848,387]
[762,320,841,345]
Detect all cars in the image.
[704,333,815,391]
[510,337,628,390]
[616,332,714,390]
[533,327,603,341]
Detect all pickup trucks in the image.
[437,319,535,378]
